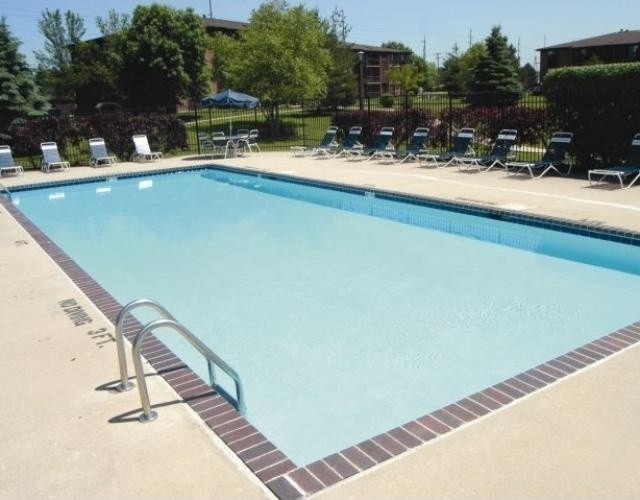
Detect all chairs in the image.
[289,125,640,190]
[0,134,165,178]
[196,128,262,160]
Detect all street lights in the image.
[405,87,423,155]
[357,51,364,109]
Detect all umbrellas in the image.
[201,85,263,139]
